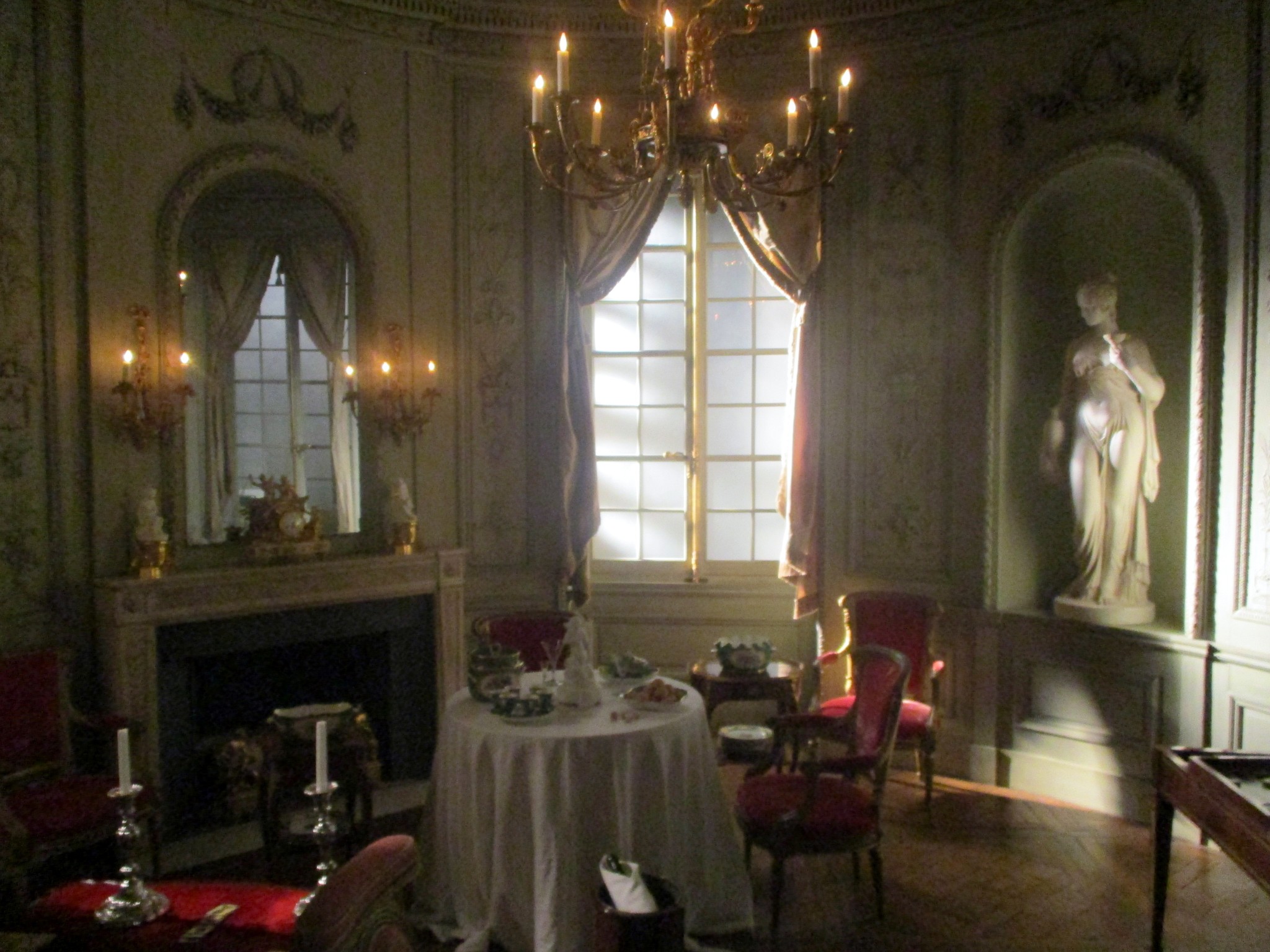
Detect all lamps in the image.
[110,304,197,451]
[342,321,441,448]
[525,0,854,216]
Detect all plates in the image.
[620,686,689,712]
[597,664,659,685]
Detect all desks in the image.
[31,879,314,952]
[689,653,804,774]
[411,668,752,952]
[255,713,379,871]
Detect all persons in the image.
[386,479,416,519]
[563,616,591,678]
[1040,272,1166,605]
[136,487,169,540]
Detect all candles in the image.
[118,728,131,793]
[316,720,327,792]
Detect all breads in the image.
[628,678,679,703]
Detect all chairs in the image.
[0,648,162,916]
[473,608,582,671]
[735,590,944,933]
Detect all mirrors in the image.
[156,141,389,565]
